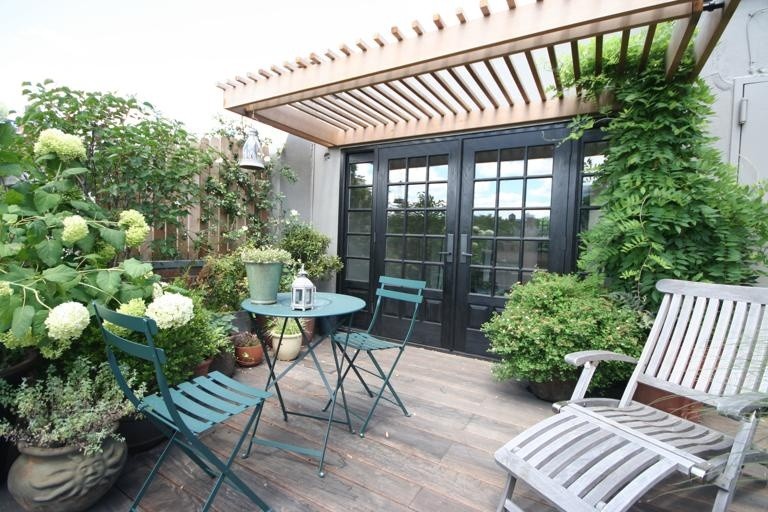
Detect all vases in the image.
[1,348,38,386]
[523,370,596,403]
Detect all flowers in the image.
[478,265,647,393]
[0,102,195,360]
[471,226,495,264]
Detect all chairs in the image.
[320,274,427,438]
[490,279,768,512]
[91,300,275,512]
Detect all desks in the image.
[240,291,367,479]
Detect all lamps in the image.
[290,262,317,312]
[236,113,266,172]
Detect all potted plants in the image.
[240,243,295,305]
[74,283,233,450]
[192,325,230,379]
[263,316,309,361]
[1,356,155,512]
[210,310,239,384]
[187,249,253,335]
[257,221,345,349]
[234,329,264,367]
[546,19,768,424]
